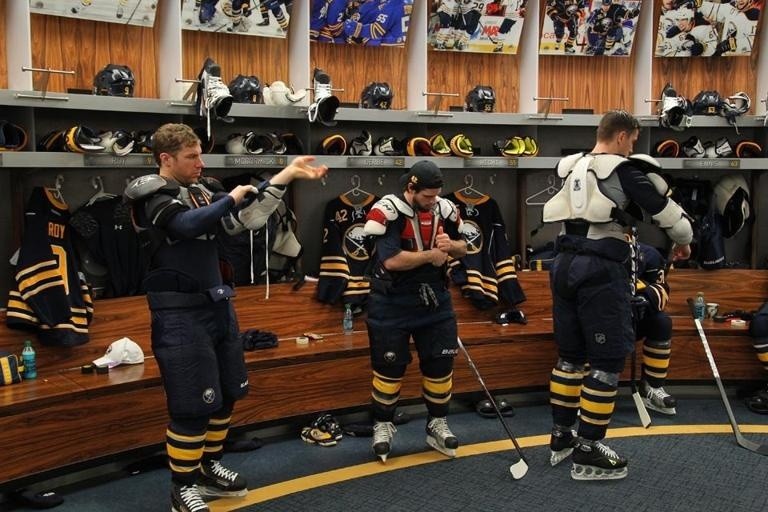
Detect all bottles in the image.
[342,303,354,335]
[20,342,37,381]
[694,291,705,324]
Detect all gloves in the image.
[345,19,363,38]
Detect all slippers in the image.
[476,397,514,418]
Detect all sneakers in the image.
[433,34,470,50]
[201,461,247,492]
[300,416,343,446]
[129,129,154,153]
[425,414,458,449]
[661,88,685,129]
[372,421,391,453]
[314,74,339,124]
[41,127,104,153]
[551,427,577,450]
[570,442,627,468]
[493,41,505,52]
[199,64,232,119]
[555,38,611,56]
[171,486,210,512]
[637,381,676,408]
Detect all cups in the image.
[705,301,720,321]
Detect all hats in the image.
[93,337,145,369]
[398,160,443,189]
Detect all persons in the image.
[366,160,467,458]
[71,0,762,57]
[630,243,677,412]
[123,122,328,512]
[745,301,767,416]
[551,109,693,471]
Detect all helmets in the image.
[463,85,496,111]
[92,65,134,97]
[360,83,394,108]
[693,90,723,115]
[227,74,262,103]
[674,7,695,20]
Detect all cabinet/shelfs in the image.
[1,88,767,170]
